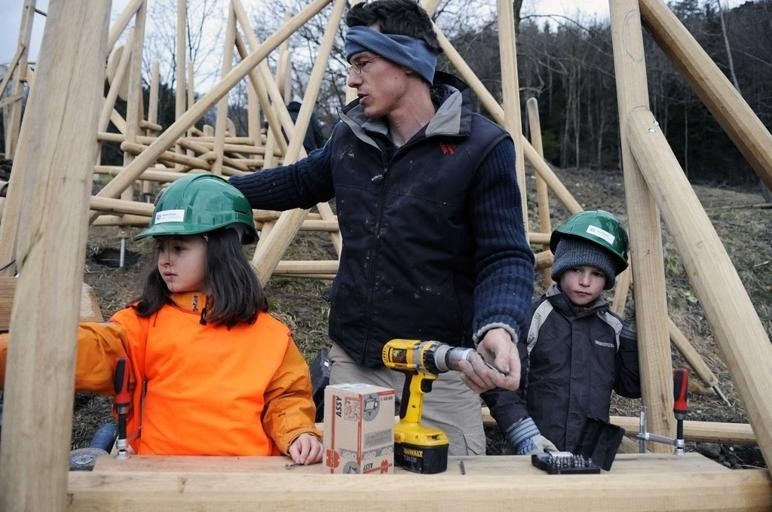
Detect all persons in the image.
[0,172,323,466]
[226,0,535,456]
[480,209,640,457]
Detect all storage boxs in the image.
[321,383,394,475]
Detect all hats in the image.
[551,234,615,290]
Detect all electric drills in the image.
[382,338,494,474]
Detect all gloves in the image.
[620,283,637,341]
[507,417,558,454]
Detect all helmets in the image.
[133,173,259,244]
[550,210,628,275]
